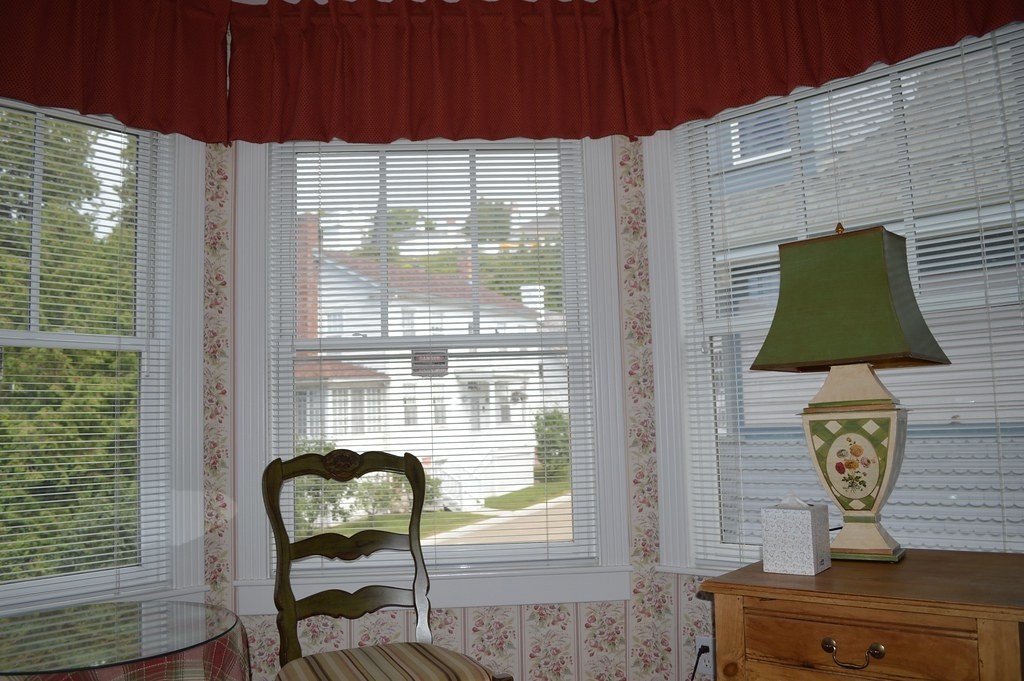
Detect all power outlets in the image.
[695,636,715,675]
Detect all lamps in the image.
[749,223,953,565]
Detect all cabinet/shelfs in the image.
[701,547,1024,681]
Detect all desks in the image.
[0,599,251,681]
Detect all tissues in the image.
[759,490,832,577]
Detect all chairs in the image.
[262,447,515,681]
